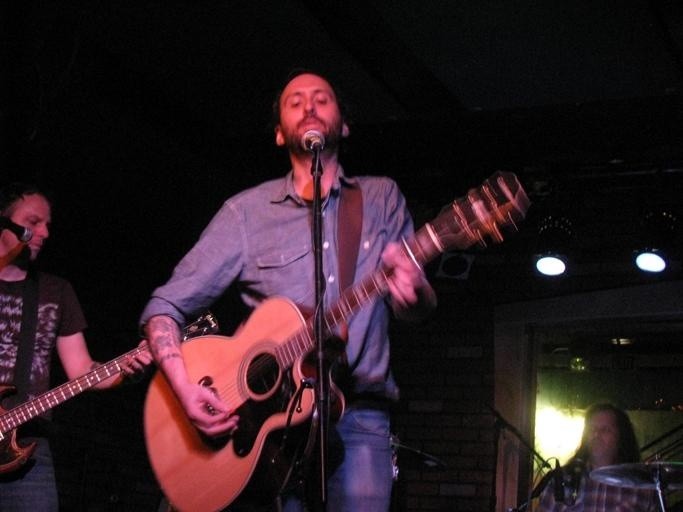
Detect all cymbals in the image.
[589,460,677,490]
[387,439,441,469]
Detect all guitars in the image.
[143,170,532,512]
[0,310,220,472]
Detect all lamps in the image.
[537,195,683,277]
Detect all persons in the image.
[0,181,156,512]
[527,400,653,511]
[137,64,438,511]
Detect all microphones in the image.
[1,215,33,242]
[554,458,565,502]
[300,128,325,152]
